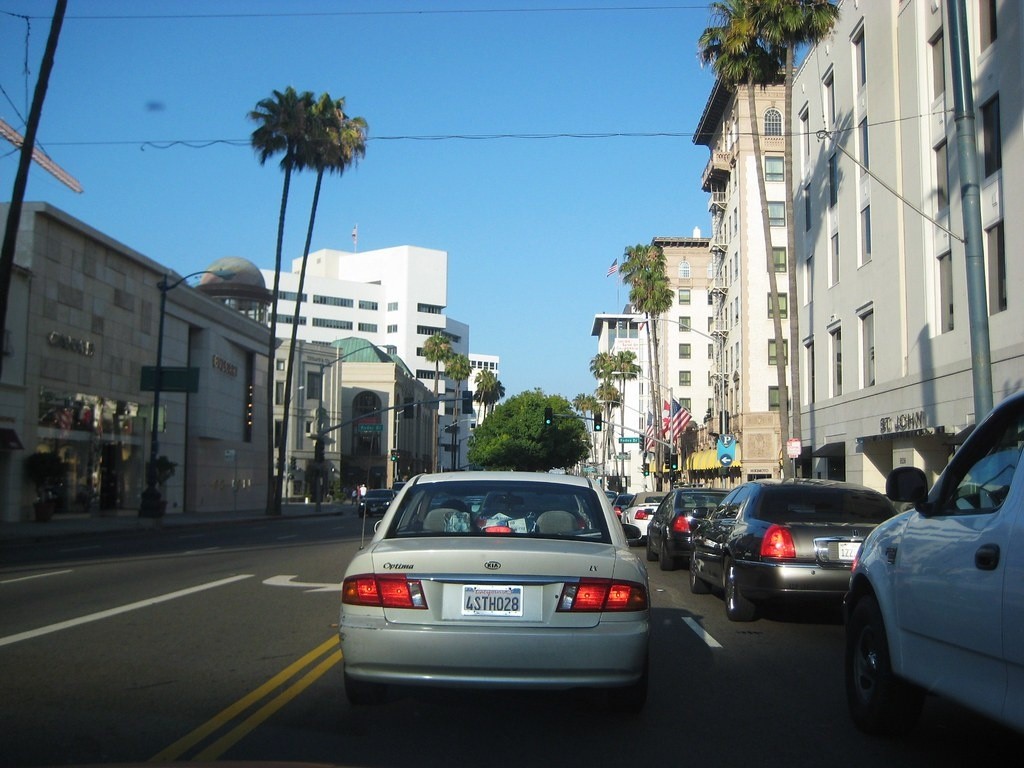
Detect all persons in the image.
[351,484,366,505]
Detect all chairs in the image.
[703,501,718,507]
[813,495,838,520]
[762,494,789,520]
[441,498,469,513]
[423,507,461,533]
[536,510,579,536]
[682,498,697,507]
[537,498,571,519]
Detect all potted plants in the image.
[139,456,179,519]
[29,448,70,522]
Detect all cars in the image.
[841,387,1024,748]
[357,488,398,518]
[601,486,697,547]
[389,481,407,494]
[326,470,654,708]
[642,486,736,570]
[687,479,900,625]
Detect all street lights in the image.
[595,400,648,454]
[440,418,477,472]
[310,345,398,512]
[631,317,726,436]
[610,370,673,456]
[137,269,236,518]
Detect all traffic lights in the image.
[671,454,678,470]
[544,407,552,425]
[594,412,602,431]
[641,463,649,477]
[390,449,396,462]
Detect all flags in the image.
[646,398,692,451]
